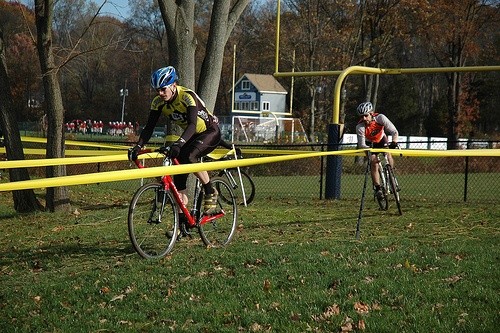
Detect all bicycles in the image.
[211,144,256,208]
[128,147,238,260]
[371,143,405,216]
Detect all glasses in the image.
[360,113,369,118]
[155,84,172,92]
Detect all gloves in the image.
[388,141,397,149]
[235,147,242,154]
[128,145,141,161]
[164,140,182,158]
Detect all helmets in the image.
[357,101,373,116]
[149,65,178,89]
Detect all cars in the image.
[154,126,165,138]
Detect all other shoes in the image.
[375,189,383,196]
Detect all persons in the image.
[66,119,103,136]
[357,102,398,200]
[107,121,139,137]
[127,65,222,238]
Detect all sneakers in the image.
[165,227,190,237]
[203,187,218,215]
